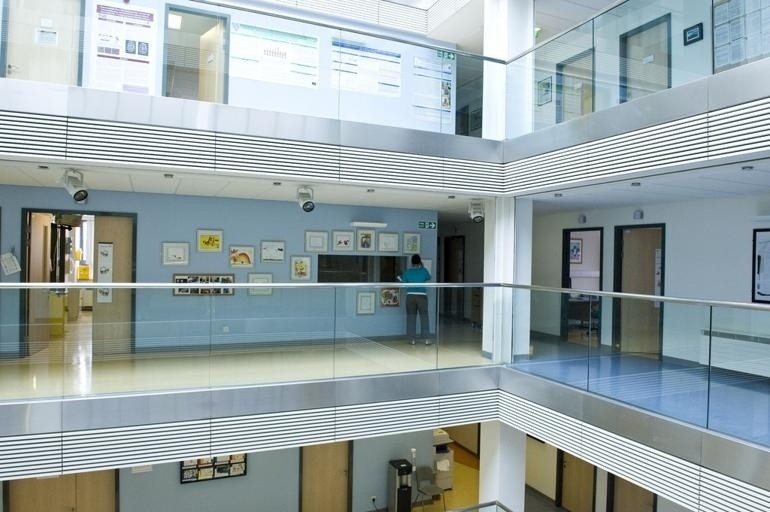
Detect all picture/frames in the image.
[289,256,312,282]
[304,228,421,254]
[537,76,552,106]
[751,226,770,303]
[160,229,286,297]
[570,238,583,264]
[356,287,400,315]
[683,22,703,46]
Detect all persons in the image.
[400,252,435,346]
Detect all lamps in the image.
[61,168,89,203]
[467,203,484,223]
[299,183,315,214]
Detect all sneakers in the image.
[411,340,415,345]
[425,339,430,345]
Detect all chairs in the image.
[413,466,446,512]
